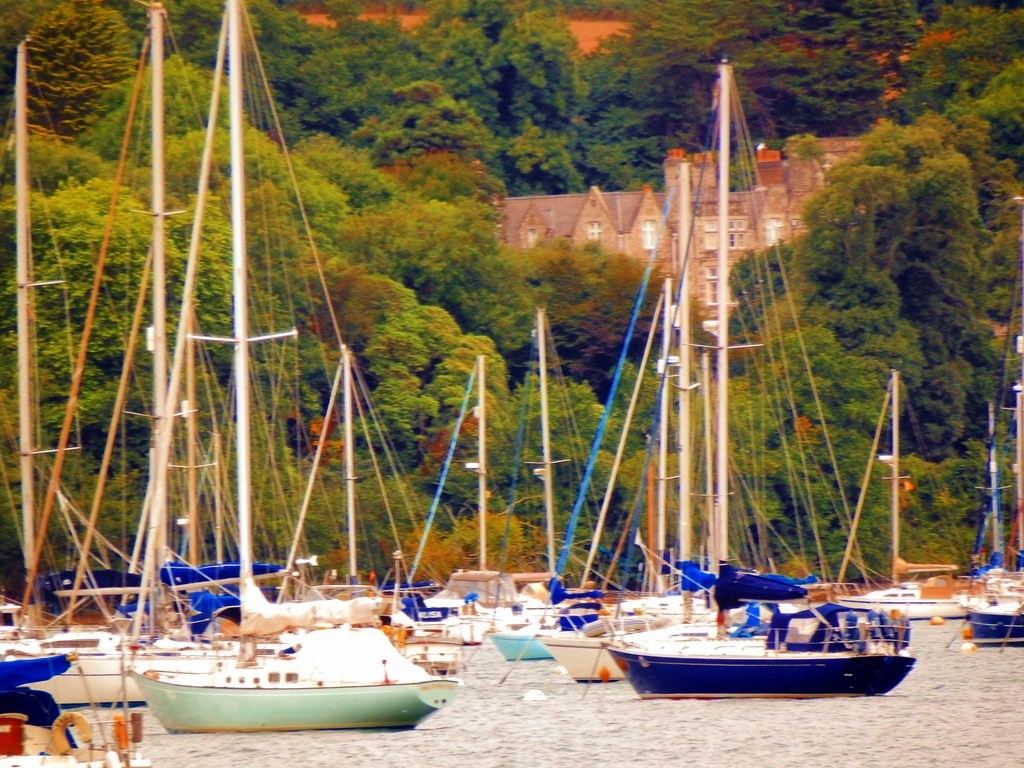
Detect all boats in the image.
[414,57,1023,698]
[0,2,493,730]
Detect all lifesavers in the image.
[51,712,92,755]
